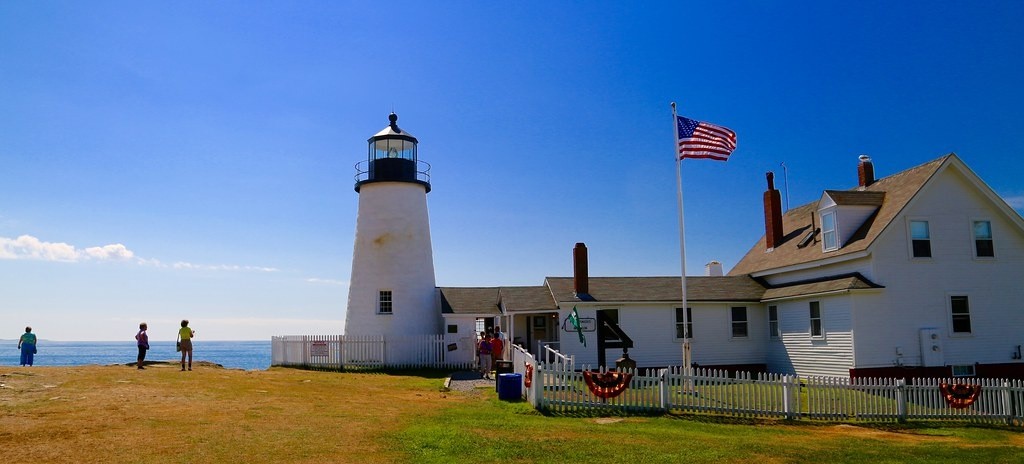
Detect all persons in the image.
[179,320,195,371]
[495,326,505,346]
[135,323,150,369]
[491,333,504,367]
[17,327,37,366]
[478,334,492,379]
[480,332,485,342]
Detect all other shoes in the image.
[138,366,145,369]
[486,375,490,379]
[181,369,186,371]
[480,373,484,378]
[188,367,192,371]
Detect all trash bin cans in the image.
[496,360,514,393]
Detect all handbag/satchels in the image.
[145,342,149,350]
[33,334,37,354]
[177,329,181,352]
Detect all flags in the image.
[569,306,583,343]
[672,114,737,162]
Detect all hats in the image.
[26,327,32,332]
[180,319,189,327]
